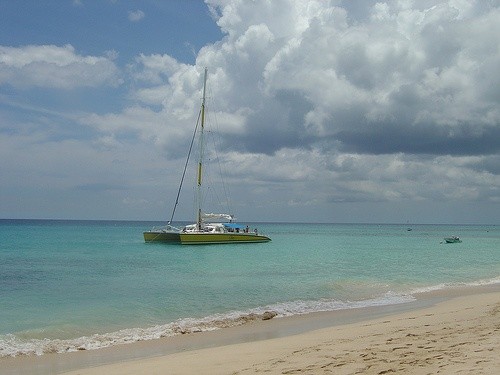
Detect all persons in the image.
[167,220,170,225]
[245,225,249,233]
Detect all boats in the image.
[142,65,274,248]
[444,235,463,244]
